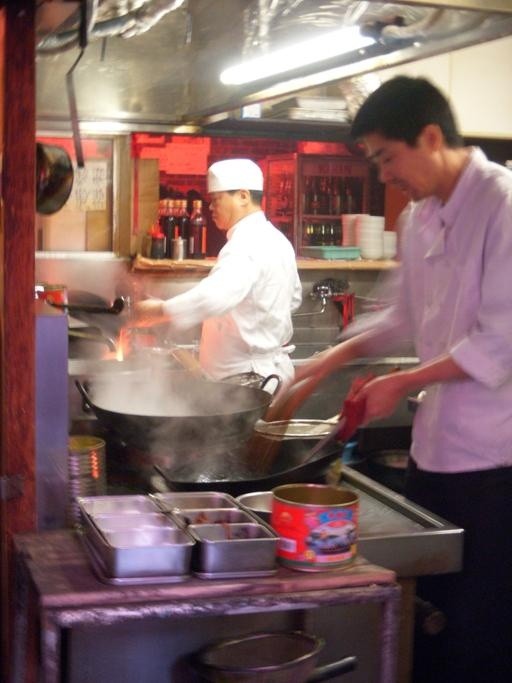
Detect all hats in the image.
[205,156,266,195]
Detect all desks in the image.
[10,527,400,683]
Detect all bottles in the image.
[303,176,353,248]
[141,199,207,260]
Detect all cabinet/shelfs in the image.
[262,153,375,255]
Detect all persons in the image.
[131,158,302,407]
[295,77,512,683]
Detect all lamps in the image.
[215,15,406,90]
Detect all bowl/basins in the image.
[339,213,397,260]
[234,490,278,525]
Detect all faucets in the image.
[293,284,331,317]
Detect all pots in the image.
[71,375,282,447]
[154,435,344,496]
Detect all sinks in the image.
[290,327,342,360]
